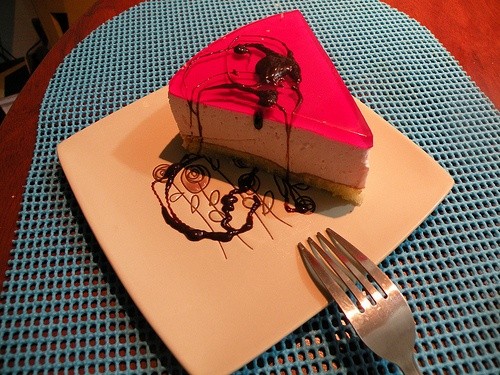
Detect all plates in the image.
[55,79,454,375]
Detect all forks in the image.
[296,228,423,375]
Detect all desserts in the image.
[167,10,374,205]
